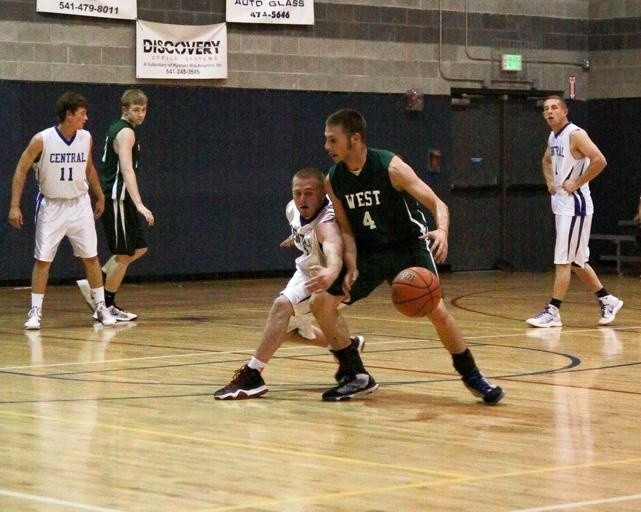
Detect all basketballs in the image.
[392,267,441,316]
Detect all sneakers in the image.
[322,370,379,401]
[214,364,269,399]
[97,307,116,326]
[597,294,624,325]
[24,307,41,330]
[461,372,505,405]
[76,278,96,311]
[92,304,138,321]
[335,335,365,383]
[525,303,562,327]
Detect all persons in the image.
[524,324,626,512]
[308,105,505,406]
[211,165,367,402]
[8,89,117,332]
[76,87,156,323]
[524,94,625,329]
[21,323,139,509]
